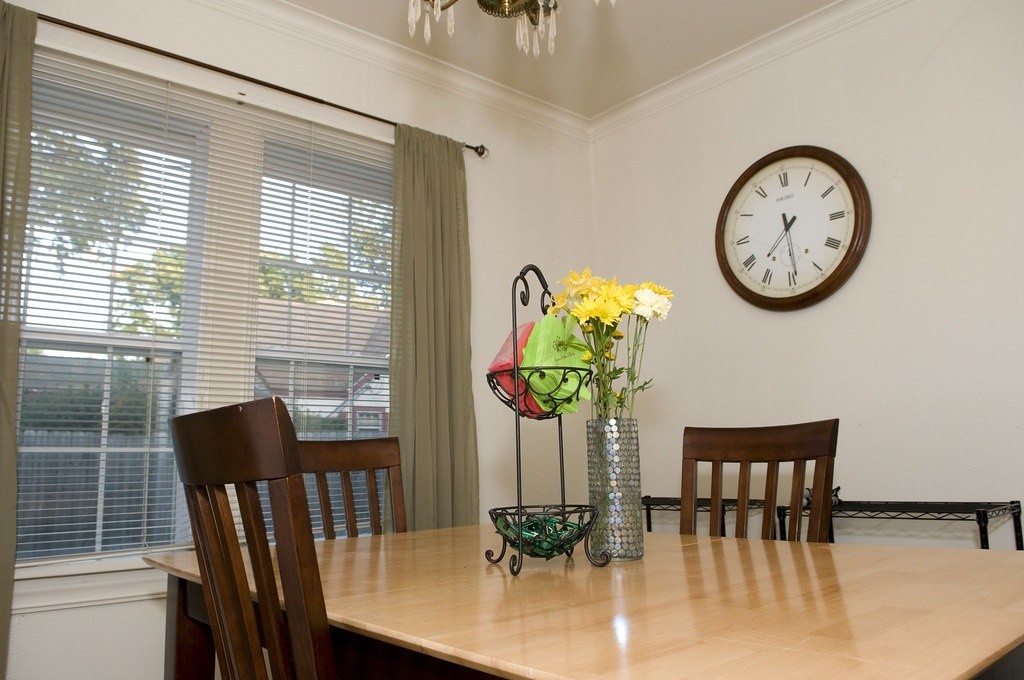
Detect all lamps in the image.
[406,0,619,58]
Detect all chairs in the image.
[167,396,340,680]
[297,437,407,539]
[679,418,841,543]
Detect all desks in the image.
[142,523,1024,680]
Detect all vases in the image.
[585,418,645,562]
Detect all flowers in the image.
[551,268,672,417]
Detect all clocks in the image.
[714,143,873,313]
